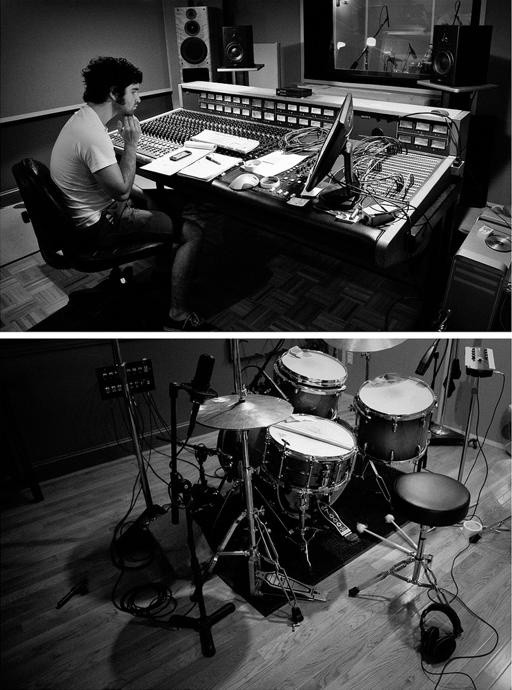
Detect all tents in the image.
[419,602,462,665]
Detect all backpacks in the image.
[260,412,357,491]
[273,348,348,419]
[351,373,437,465]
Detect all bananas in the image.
[190,129,259,154]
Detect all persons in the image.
[51,57,222,331]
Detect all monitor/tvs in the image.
[139,141,243,181]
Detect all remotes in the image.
[229,173,259,191]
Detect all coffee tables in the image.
[194,392,294,429]
[324,339,404,354]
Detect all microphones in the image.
[347,468,474,622]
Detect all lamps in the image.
[12,156,188,327]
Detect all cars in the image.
[460,520,483,539]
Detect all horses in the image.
[170,150,192,161]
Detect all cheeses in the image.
[485,234,511,251]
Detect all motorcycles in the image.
[166,384,236,658]
[109,340,171,569]
[189,430,305,623]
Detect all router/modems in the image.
[260,175,281,189]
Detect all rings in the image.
[206,156,221,165]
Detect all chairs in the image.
[163,312,220,331]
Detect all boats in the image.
[220,24,254,68]
[174,5,225,83]
[430,24,493,87]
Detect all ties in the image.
[306,93,360,209]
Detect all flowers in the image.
[414,340,441,377]
[385,5,390,27]
[189,354,215,434]
[408,42,417,59]
